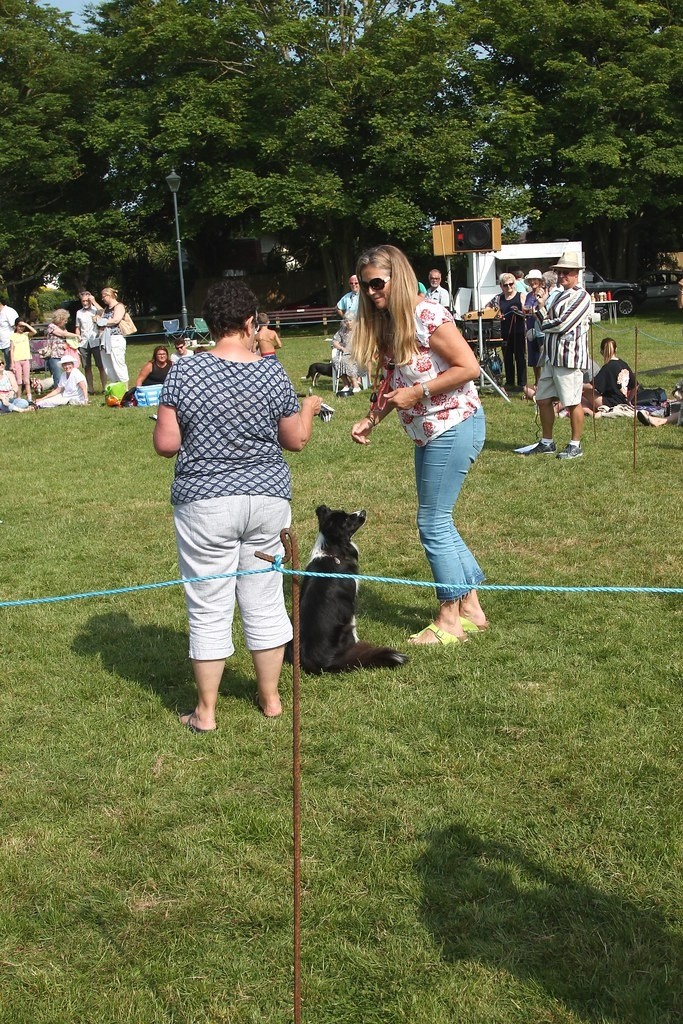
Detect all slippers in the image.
[459,616,487,634]
[407,623,469,647]
[255,691,282,718]
[180,712,216,733]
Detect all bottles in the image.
[607,291,611,300]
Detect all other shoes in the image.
[102,390,107,395]
[341,385,352,392]
[16,393,42,413]
[352,387,361,392]
[88,391,96,396]
[503,384,527,392]
[637,410,660,428]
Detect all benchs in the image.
[267,307,336,337]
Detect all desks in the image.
[466,338,503,396]
[591,301,619,324]
[30,340,46,373]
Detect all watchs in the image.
[422,382,431,398]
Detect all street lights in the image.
[165,165,189,338]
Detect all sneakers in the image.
[555,444,583,460]
[529,440,556,455]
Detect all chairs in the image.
[332,343,368,392]
[163,318,213,346]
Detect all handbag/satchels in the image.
[105,381,162,407]
[38,345,52,359]
[118,312,137,337]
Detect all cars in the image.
[585,267,683,317]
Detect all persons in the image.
[33,355,88,408]
[523,337,683,428]
[44,309,81,390]
[335,275,360,316]
[194,346,208,354]
[426,270,450,310]
[515,271,531,293]
[351,247,490,645]
[417,282,426,295]
[535,271,563,349]
[76,291,107,395]
[252,313,282,358]
[170,338,194,365]
[136,346,175,386]
[525,251,591,458]
[153,279,324,732]
[485,272,526,391]
[92,287,130,391]
[0,295,37,413]
[583,357,601,383]
[521,270,543,386]
[332,311,367,392]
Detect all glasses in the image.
[503,281,514,287]
[557,270,578,275]
[175,345,184,350]
[349,282,359,285]
[359,274,392,292]
[0,361,4,366]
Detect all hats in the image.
[524,269,543,286]
[550,251,586,270]
[57,355,78,369]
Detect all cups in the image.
[193,340,197,345]
[591,294,607,301]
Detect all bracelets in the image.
[366,416,376,426]
[85,398,88,401]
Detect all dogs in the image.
[301,363,342,387]
[283,505,411,677]
